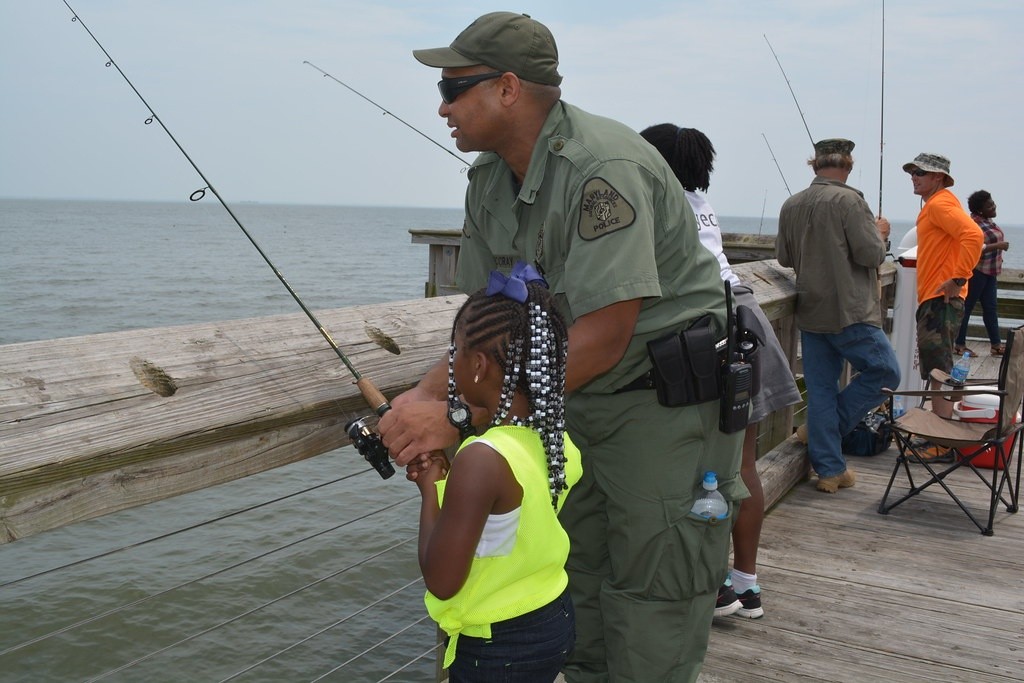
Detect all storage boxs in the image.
[953,385,1022,469]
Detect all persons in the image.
[638,123,803,619]
[378,11,765,683]
[406,260,582,683]
[953,191,1010,357]
[776,139,900,494]
[903,152,984,463]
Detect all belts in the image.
[613,368,655,392]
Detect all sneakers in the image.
[817,468,855,493]
[797,424,808,444]
[736,585,764,619]
[713,573,744,617]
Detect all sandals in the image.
[990,347,1004,356]
[907,444,955,464]
[954,347,976,357]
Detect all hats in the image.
[413,11,564,87]
[902,152,954,188]
[814,138,855,155]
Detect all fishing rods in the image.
[761,32,815,144]
[61,0,396,482]
[300,58,472,176]
[876,0,886,300]
[761,132,910,465]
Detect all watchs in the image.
[951,277,967,287]
[447,398,473,444]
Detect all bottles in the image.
[892,395,905,419]
[690,471,730,521]
[950,352,970,383]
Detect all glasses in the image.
[437,71,502,104]
[909,169,928,177]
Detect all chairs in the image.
[876,325,1024,538]
